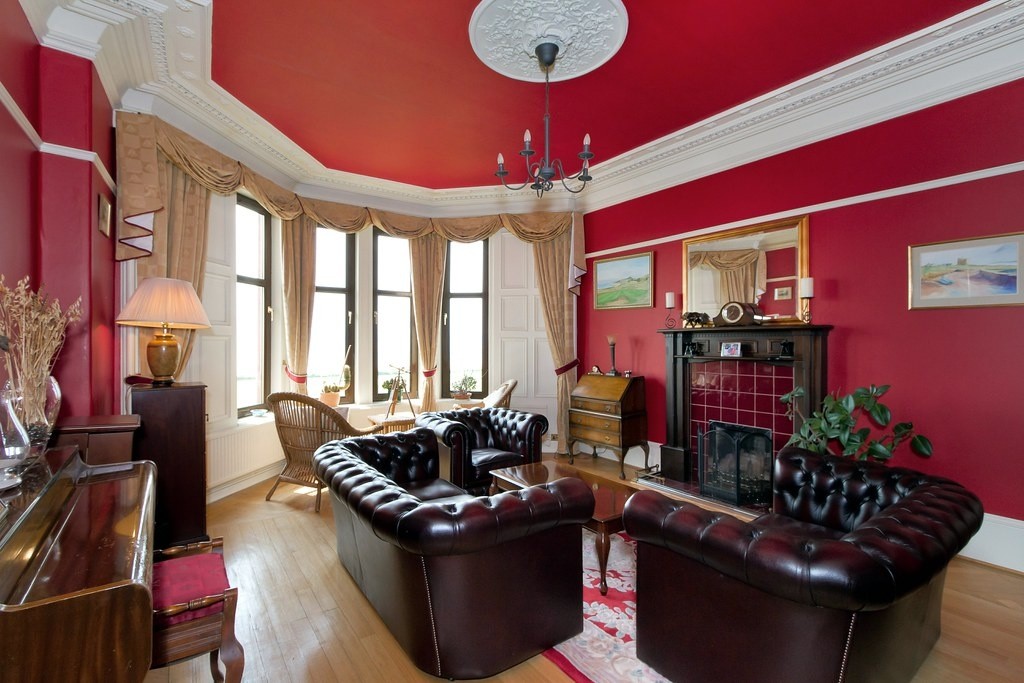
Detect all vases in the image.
[0,377,62,459]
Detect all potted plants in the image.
[452,376,477,400]
[383,378,406,403]
[320,365,351,408]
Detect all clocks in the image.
[711,302,763,326]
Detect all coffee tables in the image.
[489,461,641,597]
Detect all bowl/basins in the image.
[250,409,268,416]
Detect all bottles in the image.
[0,389,31,490]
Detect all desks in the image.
[370,412,420,426]
[49,411,141,466]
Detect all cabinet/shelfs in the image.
[132,380,208,548]
[567,375,650,480]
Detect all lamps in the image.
[493,41,595,199]
[113,277,212,387]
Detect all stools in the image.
[149,538,245,683]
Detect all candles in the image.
[800,278,813,298]
[664,292,675,309]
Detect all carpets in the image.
[541,525,675,683]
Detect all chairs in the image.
[451,378,517,409]
[263,393,385,514]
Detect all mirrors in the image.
[682,213,810,328]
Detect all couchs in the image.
[623,446,984,682]
[310,425,596,681]
[419,408,550,495]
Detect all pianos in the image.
[0,446,158,683]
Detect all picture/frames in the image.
[906,230,1024,310]
[593,249,655,311]
